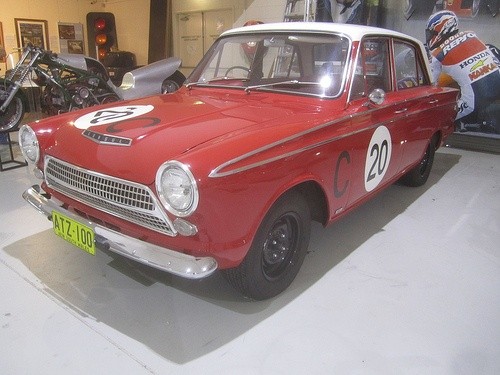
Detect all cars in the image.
[17,21,463,301]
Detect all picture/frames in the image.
[58,25,83,54]
[15,18,50,60]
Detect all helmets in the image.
[425,10,460,51]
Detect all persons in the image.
[425,10,500,123]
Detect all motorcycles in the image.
[0,36,188,136]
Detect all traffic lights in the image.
[86,11,119,64]
